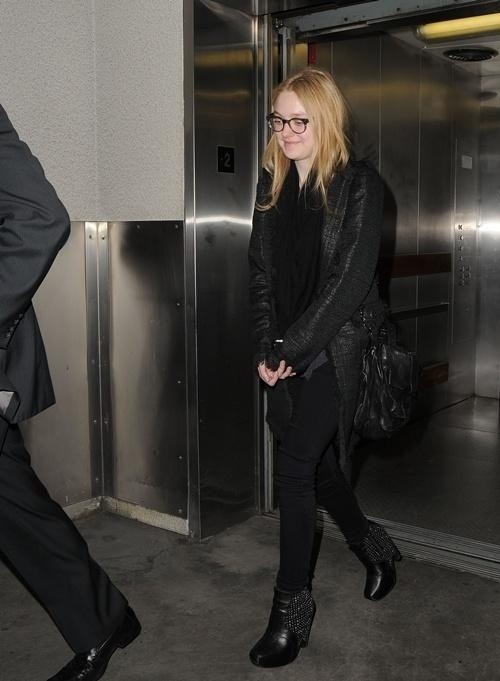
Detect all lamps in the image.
[413,12,500,43]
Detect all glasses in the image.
[266,113,310,134]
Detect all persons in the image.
[0,106,141,681]
[248,68,403,667]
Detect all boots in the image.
[348,518,403,601]
[249,586,316,668]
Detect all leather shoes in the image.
[47,605,141,681]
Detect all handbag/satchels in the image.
[353,343,422,442]
